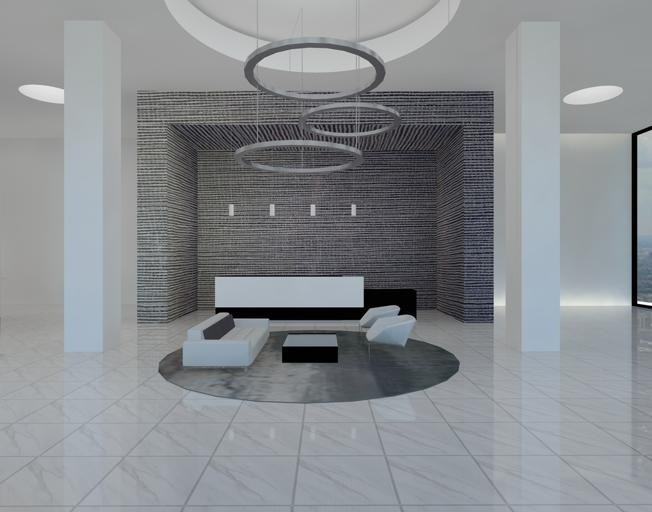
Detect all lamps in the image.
[226,0,405,220]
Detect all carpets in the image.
[158,331,460,405]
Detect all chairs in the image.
[358,304,401,336]
[365,314,417,360]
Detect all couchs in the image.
[181,312,271,372]
